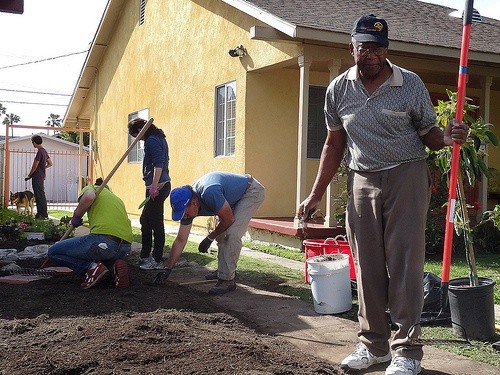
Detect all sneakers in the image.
[139,258,164,270]
[385,356,421,375]
[205,270,219,280]
[113,259,128,288]
[212,279,235,294]
[340,342,391,371]
[80,262,109,289]
[132,257,149,268]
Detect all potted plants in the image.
[424,87,500,338]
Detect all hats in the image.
[170,187,192,221]
[351,13,388,46]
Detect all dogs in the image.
[9,189,35,217]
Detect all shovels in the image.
[138,196,151,209]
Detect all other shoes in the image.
[34,213,48,219]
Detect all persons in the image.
[128,117,172,269]
[25,134,53,218]
[48,175,133,288]
[298,15,468,375]
[153,171,266,292]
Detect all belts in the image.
[246,177,253,189]
[106,235,130,244]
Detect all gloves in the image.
[198,235,213,252]
[71,216,83,227]
[153,271,168,286]
[149,186,159,200]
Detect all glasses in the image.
[353,43,387,57]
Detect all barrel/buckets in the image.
[303,239,357,285]
[306,253,352,315]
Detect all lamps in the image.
[229,45,246,58]
